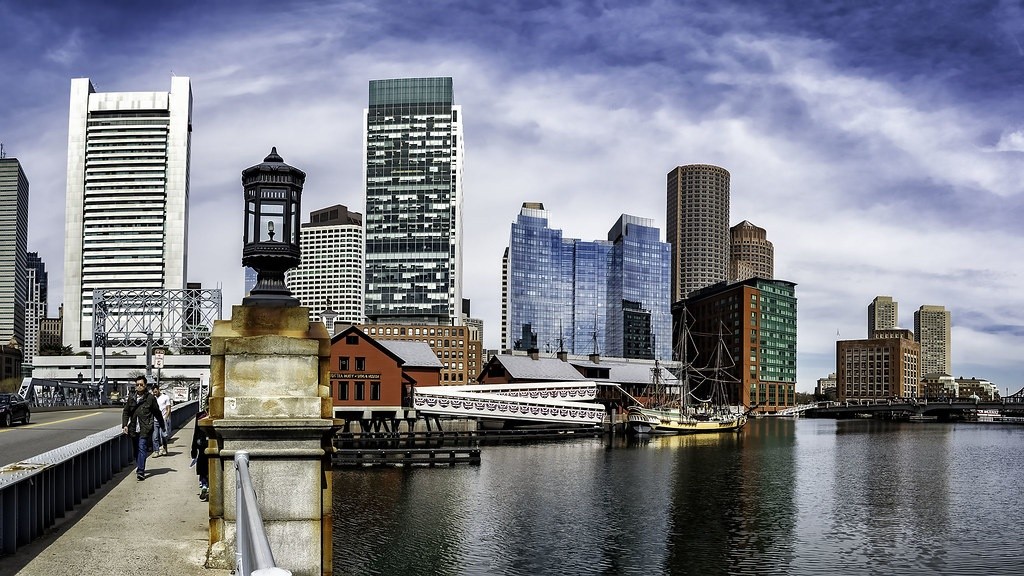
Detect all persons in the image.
[120,375,166,480]
[191,393,210,500]
[153,385,171,457]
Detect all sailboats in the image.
[627,302,748,436]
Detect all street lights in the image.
[796,402,798,407]
[913,396,915,412]
[895,396,897,403]
[200,373,203,411]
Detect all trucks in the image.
[172,387,190,405]
[111,392,119,402]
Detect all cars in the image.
[993,399,1002,402]
[0,393,31,426]
[944,393,956,401]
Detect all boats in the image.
[968,410,1006,418]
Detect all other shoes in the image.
[152,452,159,457]
[162,447,167,455]
[199,486,209,501]
[136,474,145,480]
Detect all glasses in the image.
[136,383,143,386]
